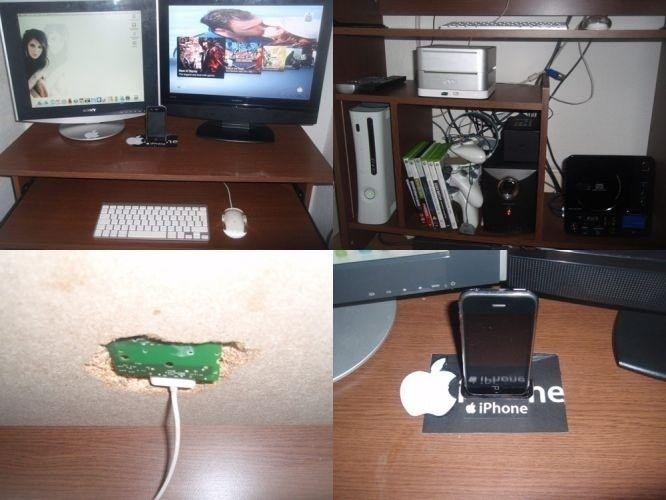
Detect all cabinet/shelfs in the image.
[333,0,666,249]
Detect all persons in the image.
[177,8,312,51]
[20,27,52,100]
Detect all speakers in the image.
[498,117,539,165]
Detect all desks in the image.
[334,289,666,499]
[0,121,336,248]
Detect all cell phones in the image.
[146,105,167,144]
[457,288,539,400]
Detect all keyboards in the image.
[91,202,211,244]
[439,19,569,30]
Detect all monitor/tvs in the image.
[507,249,666,383]
[0,0,160,142]
[333,249,507,383]
[159,1,334,144]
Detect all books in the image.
[403,138,458,229]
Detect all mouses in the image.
[221,207,248,239]
[581,16,612,31]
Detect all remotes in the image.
[337,75,406,95]
